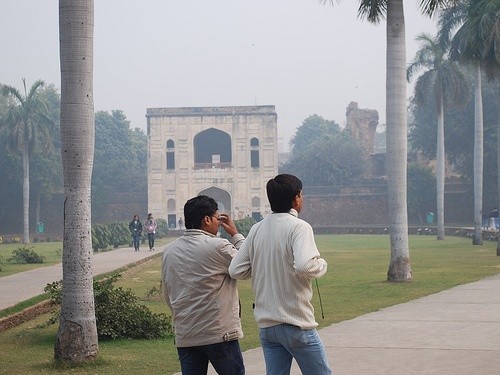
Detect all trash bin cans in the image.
[36,222,43,233]
[425,212,435,226]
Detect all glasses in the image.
[212,216,222,221]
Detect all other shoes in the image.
[149,249,154,251]
[135,248,139,251]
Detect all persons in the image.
[144,213,157,252]
[129,215,143,251]
[228,173,332,375]
[483,208,500,230]
[178,218,185,231]
[160,195,246,375]
